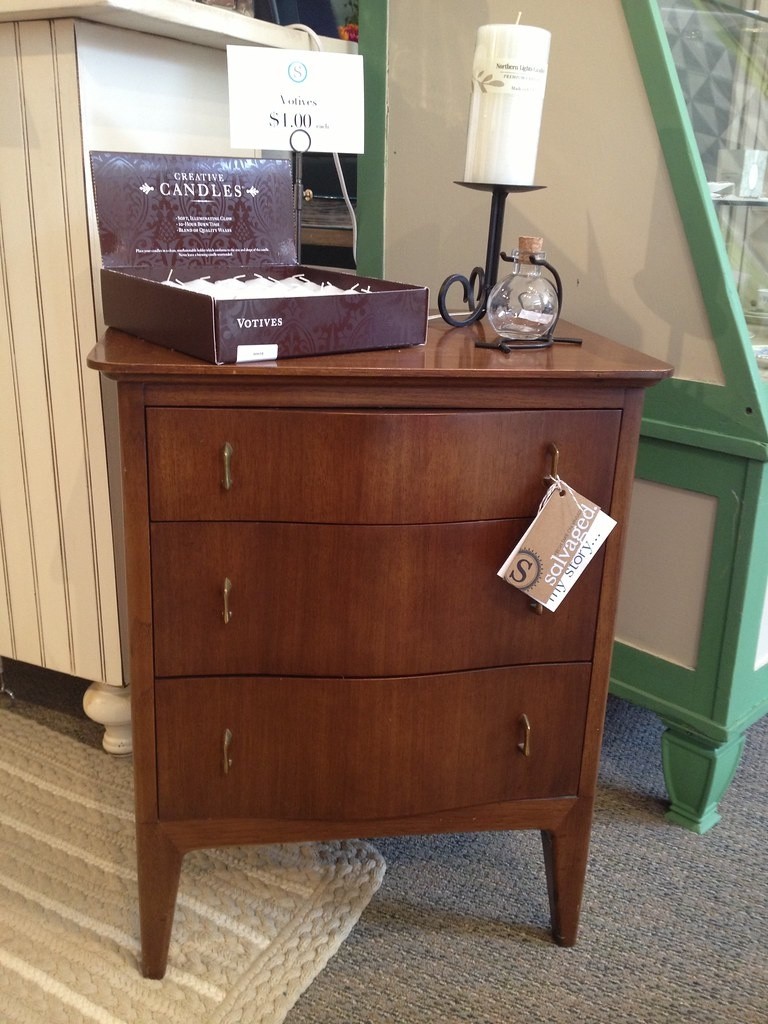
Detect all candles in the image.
[462,12,553,187]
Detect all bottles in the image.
[487,236,558,341]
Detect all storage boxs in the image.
[89,148,430,367]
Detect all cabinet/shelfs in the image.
[0,0,358,754]
[84,303,677,982]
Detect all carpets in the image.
[0,693,388,1024]
